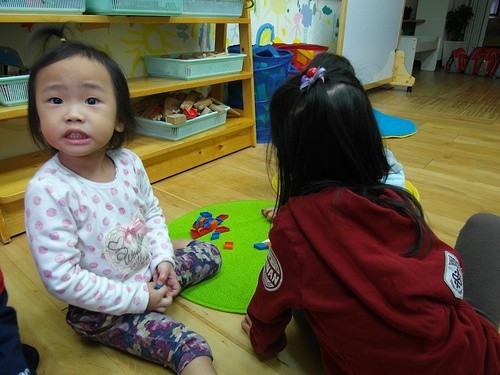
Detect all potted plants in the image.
[443,4,474,72]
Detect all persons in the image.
[0,269,41,375]
[245,66,500,375]
[26,43,222,375]
[260,53,406,222]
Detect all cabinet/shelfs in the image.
[399,19,441,72]
[0,0,257,244]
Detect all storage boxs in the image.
[141,51,247,80]
[0,0,244,18]
[0,75,28,106]
[134,105,226,140]
